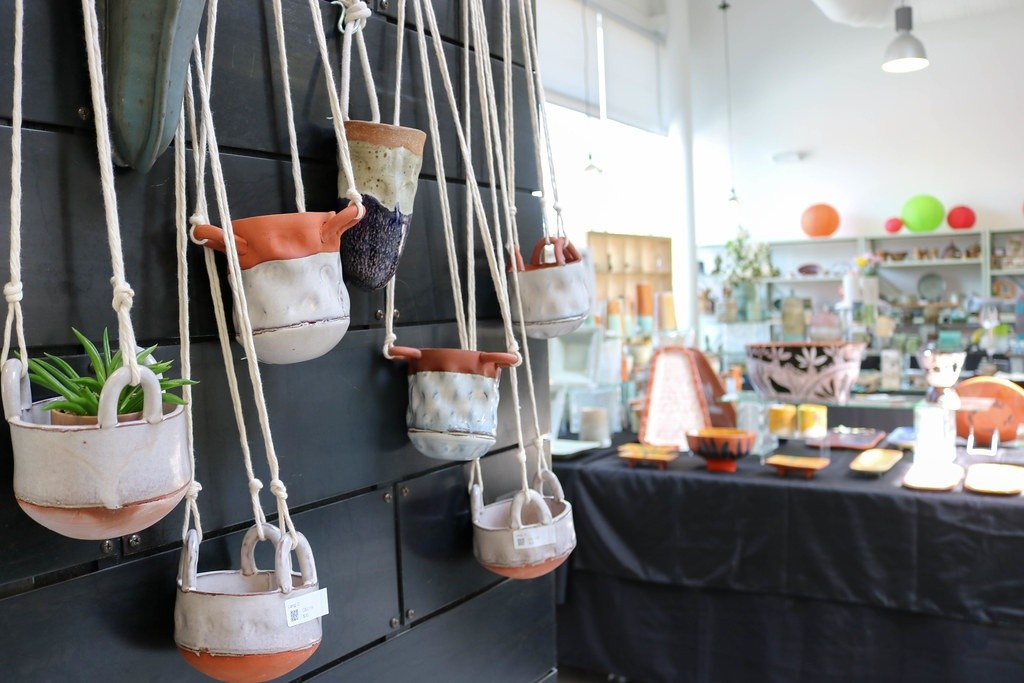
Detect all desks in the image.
[551,433,1024,683]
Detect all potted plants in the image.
[14,324,201,426]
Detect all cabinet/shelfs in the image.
[696,229,1023,354]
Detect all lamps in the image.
[880,0,929,75]
